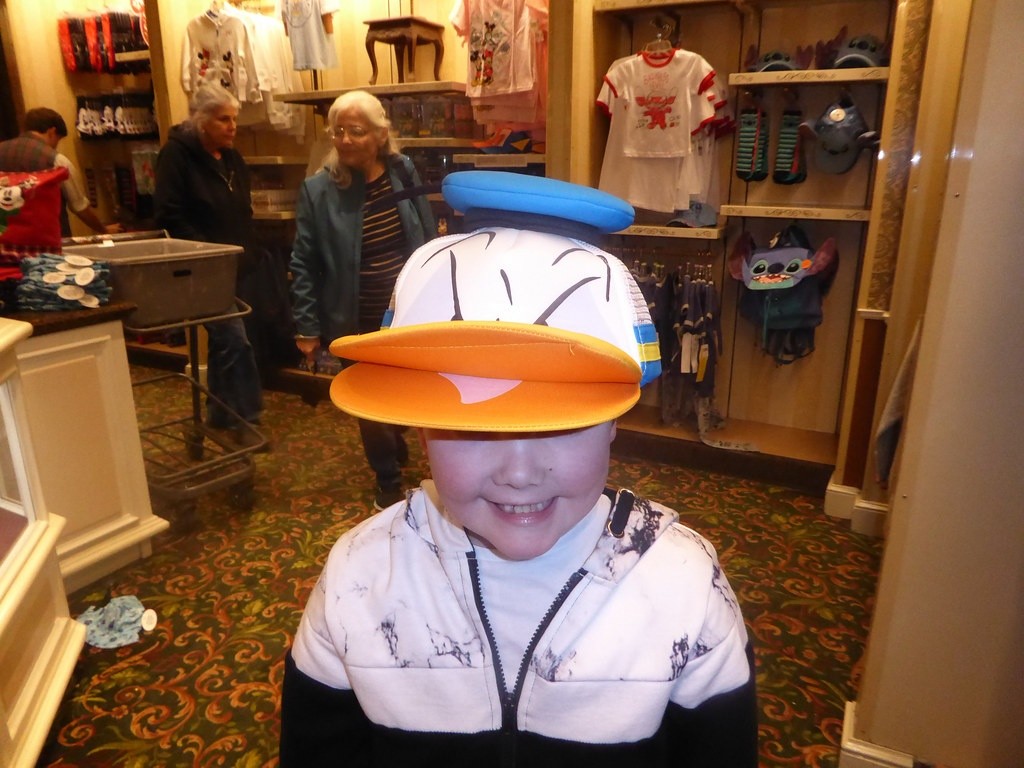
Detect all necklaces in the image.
[214,167,235,192]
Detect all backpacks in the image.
[250,244,306,364]
[727,219,839,365]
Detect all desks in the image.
[0,299,170,597]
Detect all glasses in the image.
[334,126,377,137]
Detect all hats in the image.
[756,50,796,72]
[76,594,157,650]
[328,227,665,432]
[833,35,889,67]
[473,126,533,154]
[665,201,717,227]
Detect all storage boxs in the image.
[61,238,245,328]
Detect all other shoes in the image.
[205,418,269,452]
[373,481,401,511]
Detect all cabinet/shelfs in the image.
[571,0,906,485]
[243,81,546,220]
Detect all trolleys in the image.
[59,229,272,533]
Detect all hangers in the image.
[605,246,713,282]
[640,23,672,58]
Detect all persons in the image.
[151,83,277,456]
[282,89,440,512]
[0,107,123,284]
[278,225,762,768]
